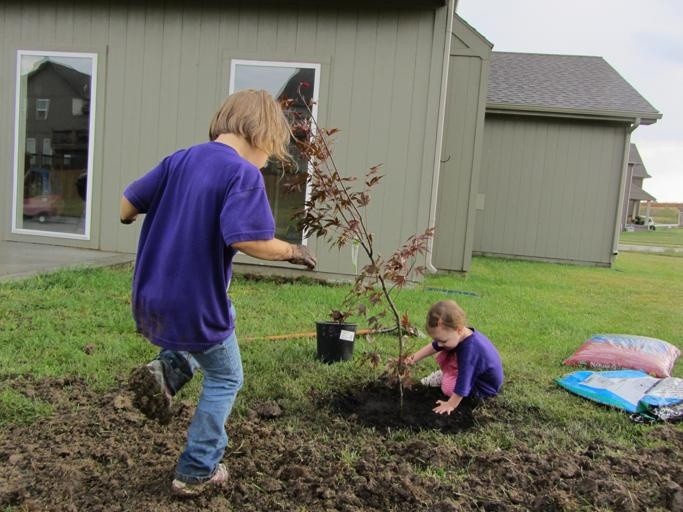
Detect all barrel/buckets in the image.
[316,322,357,362]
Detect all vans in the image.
[631,215,654,226]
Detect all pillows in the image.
[559,332,682,379]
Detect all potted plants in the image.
[314,305,360,366]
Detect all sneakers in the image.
[171,464,228,497]
[142,358,173,407]
[421,369,441,387]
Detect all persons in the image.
[403,300,502,416]
[116,88,316,499]
